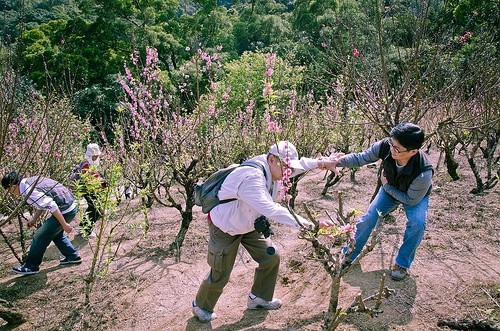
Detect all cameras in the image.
[254,215,274,239]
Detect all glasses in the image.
[387,140,408,153]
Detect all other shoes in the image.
[75,226,89,240]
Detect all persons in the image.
[124,186,132,198]
[191,141,347,322]
[318,123,434,282]
[2,172,82,274]
[73,142,109,236]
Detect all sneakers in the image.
[12,263,40,274]
[192,301,217,323]
[60,256,82,264]
[391,263,406,281]
[247,293,282,310]
[329,260,351,276]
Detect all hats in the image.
[270,141,306,171]
[87,143,101,156]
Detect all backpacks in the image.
[69,161,90,197]
[196,159,267,214]
